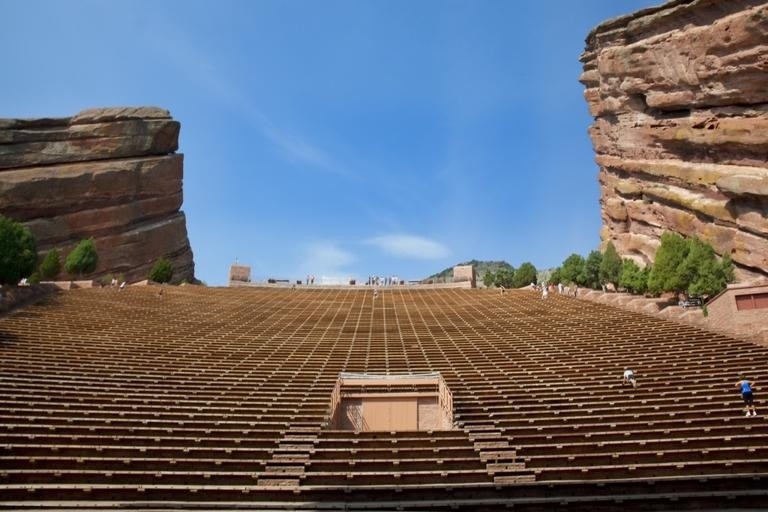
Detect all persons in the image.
[500,285,505,292]
[531,281,564,301]
[119,280,126,290]
[623,367,638,388]
[156,288,164,301]
[112,276,117,288]
[305,274,315,284]
[735,375,757,417]
[368,273,399,299]
